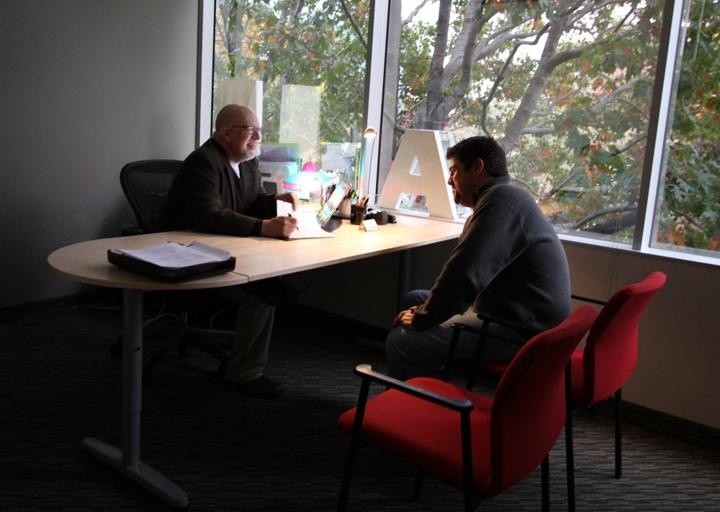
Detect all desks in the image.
[44,207,474,508]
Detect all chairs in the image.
[111,158,233,378]
[454,270,669,512]
[333,307,602,512]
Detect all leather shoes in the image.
[234,375,283,400]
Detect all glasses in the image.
[232,125,261,134]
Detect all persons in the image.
[164,105,298,398]
[382,136,571,378]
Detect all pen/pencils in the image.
[356,196,369,208]
[327,184,357,199]
[288,213,299,231]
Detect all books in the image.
[275,199,335,239]
[108,239,237,284]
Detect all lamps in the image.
[364,128,378,201]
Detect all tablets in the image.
[316,179,352,228]
